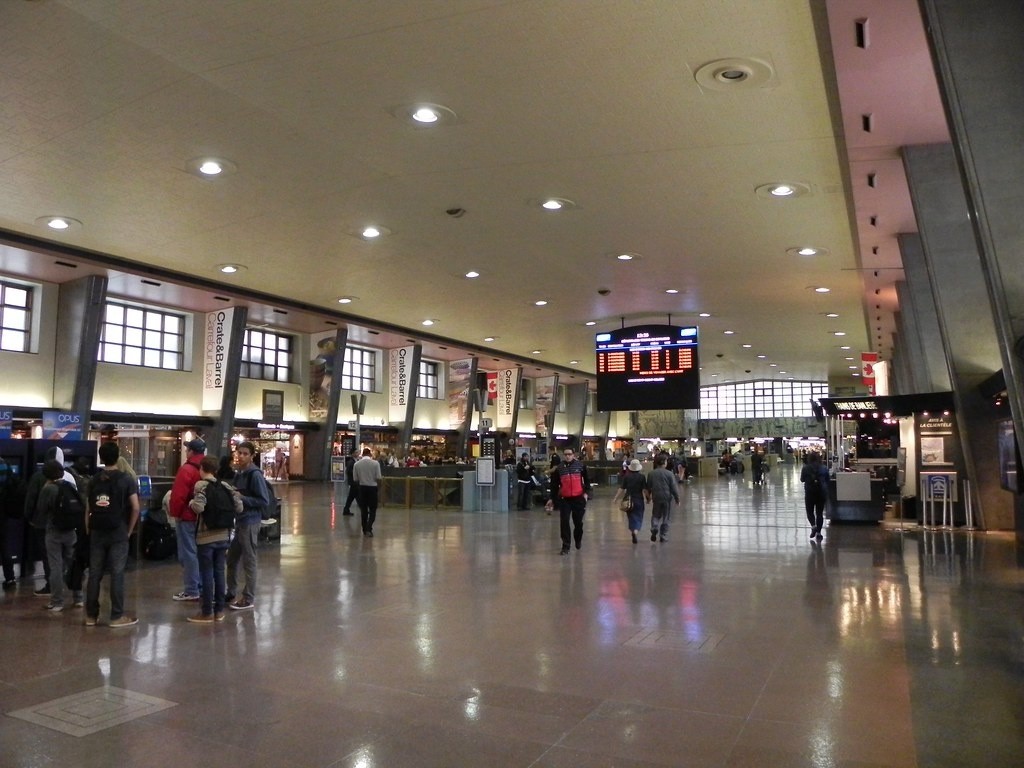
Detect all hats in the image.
[628,459,642,471]
[183,439,207,453]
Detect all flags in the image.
[860,352,880,395]
[487,371,498,398]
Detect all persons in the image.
[646,454,680,542]
[722,449,762,485]
[549,447,591,555]
[343,448,371,515]
[0,438,269,628]
[516,453,536,511]
[353,450,382,537]
[377,450,517,467]
[545,446,561,511]
[800,451,830,541]
[612,459,651,543]
[620,454,630,474]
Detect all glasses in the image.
[565,453,572,456]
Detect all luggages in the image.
[140,509,176,557]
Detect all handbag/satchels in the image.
[619,494,635,513]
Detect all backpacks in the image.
[246,469,275,520]
[50,480,87,534]
[202,479,238,530]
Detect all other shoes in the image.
[42,600,64,612]
[211,594,237,604]
[575,539,581,550]
[215,611,226,621]
[650,528,658,542]
[85,617,100,625]
[660,535,669,542]
[2,580,17,587]
[343,512,354,516]
[109,616,140,627]
[364,529,373,536]
[173,592,200,601]
[187,612,214,622]
[72,590,85,607]
[560,543,570,555]
[33,588,52,597]
[632,529,639,543]
[229,601,255,610]
